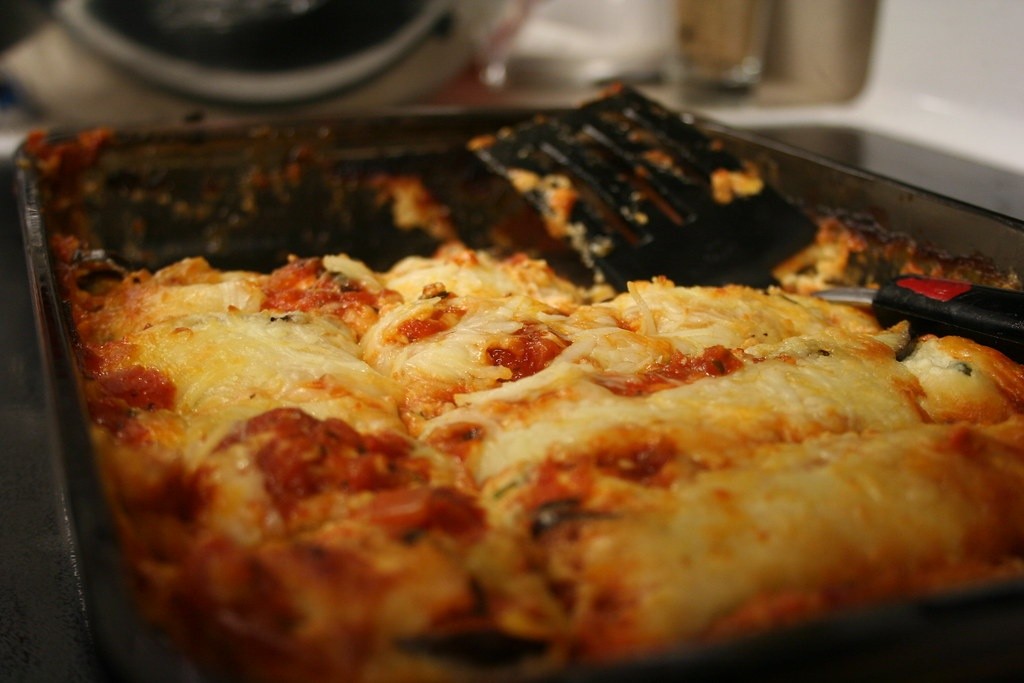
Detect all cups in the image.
[674,0,770,96]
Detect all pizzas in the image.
[60,238,1024,683]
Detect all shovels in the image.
[462,79,1023,362]
